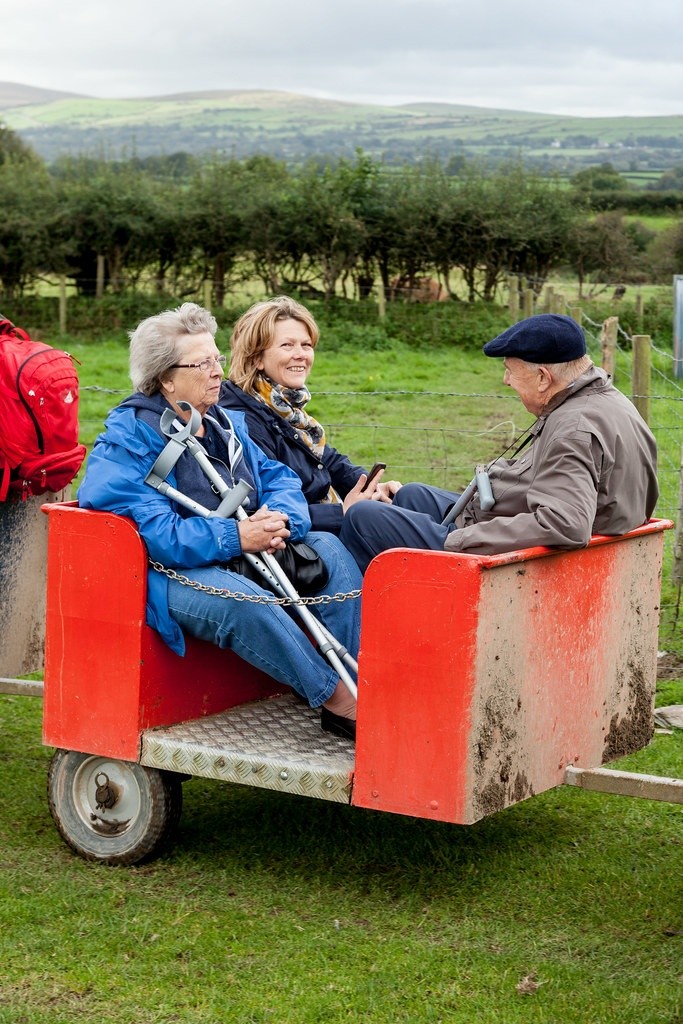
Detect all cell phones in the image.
[361,462,386,493]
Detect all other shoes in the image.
[321,705,356,741]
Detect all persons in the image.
[75,301,362,739]
[227,293,402,514]
[336,313,659,579]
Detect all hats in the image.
[483,315,586,363]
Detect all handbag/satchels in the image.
[221,511,329,598]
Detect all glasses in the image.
[170,355,226,372]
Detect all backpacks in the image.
[0,319,87,503]
